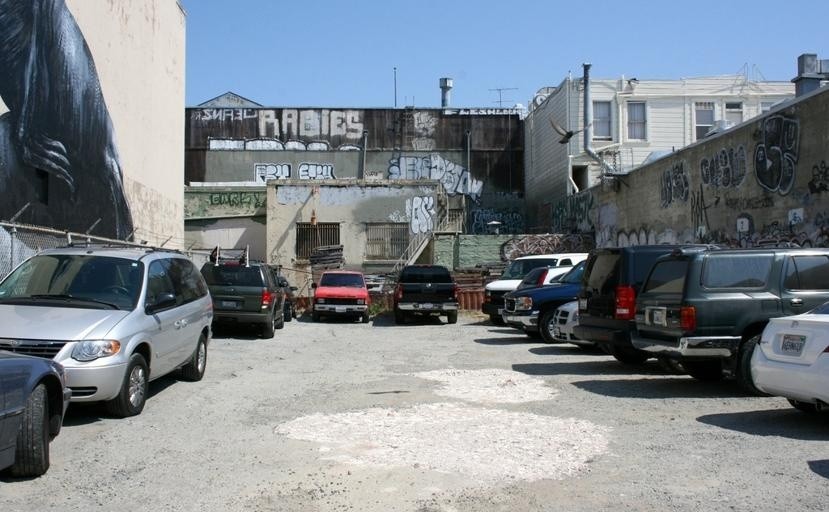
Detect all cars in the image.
[393,265,460,324]
[311,271,371,322]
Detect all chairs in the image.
[121,269,154,305]
